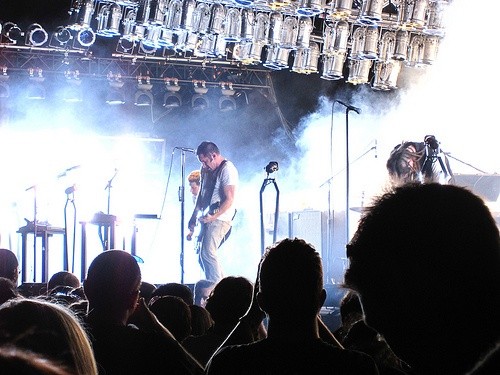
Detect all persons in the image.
[185,141,239,282]
[386,140,454,185]
[0,183,500,375]
[186,169,237,272]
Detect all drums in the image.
[92,213,118,226]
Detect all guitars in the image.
[191,201,221,254]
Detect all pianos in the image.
[16,217,66,234]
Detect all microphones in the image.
[175,147,197,154]
[375,139,377,158]
[336,99,362,114]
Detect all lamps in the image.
[0,0,446,113]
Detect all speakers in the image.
[17,282,47,298]
